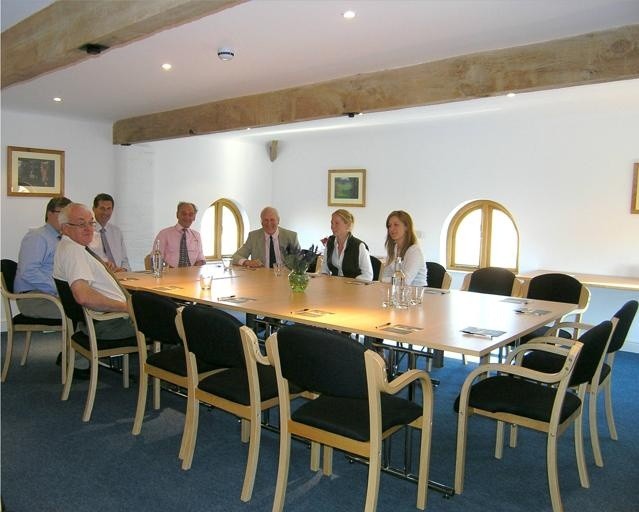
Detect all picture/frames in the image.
[7,146,65,197]
[630,163,639,214]
[328,169,365,207]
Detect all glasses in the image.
[100,228,117,268]
[65,222,97,228]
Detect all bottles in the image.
[391,257,405,305]
[151,239,161,276]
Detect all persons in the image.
[367,211,428,359]
[320,209,374,282]
[153,202,206,268]
[53,203,137,341]
[12,197,91,380]
[85,194,132,272]
[231,207,302,337]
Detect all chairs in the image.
[128,291,251,444]
[455,318,615,512]
[1,259,68,384]
[425,262,451,290]
[271,327,433,511]
[523,273,591,341]
[369,255,381,282]
[460,267,522,297]
[543,300,639,468]
[181,303,321,502]
[52,274,161,422]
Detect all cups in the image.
[383,286,413,309]
[200,273,212,289]
[273,263,281,277]
[199,289,211,303]
[223,268,233,279]
[416,285,424,305]
[222,253,234,267]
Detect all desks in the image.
[115,265,580,497]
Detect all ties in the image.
[178,229,188,267]
[270,236,276,268]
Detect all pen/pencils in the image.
[376,323,391,328]
[291,309,309,314]
[218,296,235,299]
